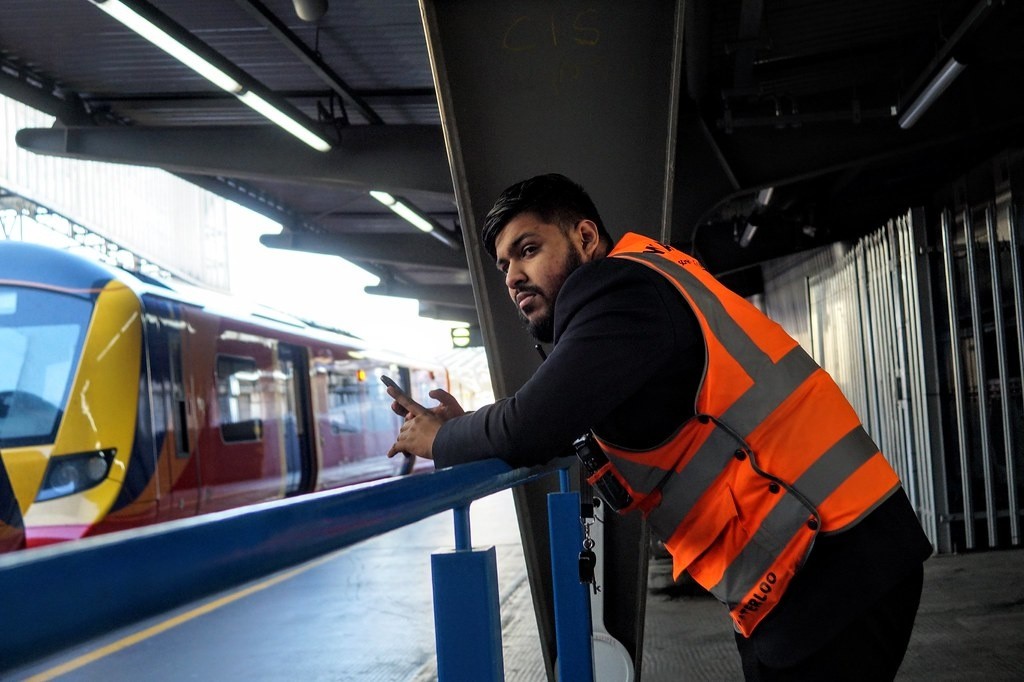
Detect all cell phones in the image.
[381,375,404,394]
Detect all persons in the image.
[388,172,934,682]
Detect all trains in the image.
[0,235,477,555]
